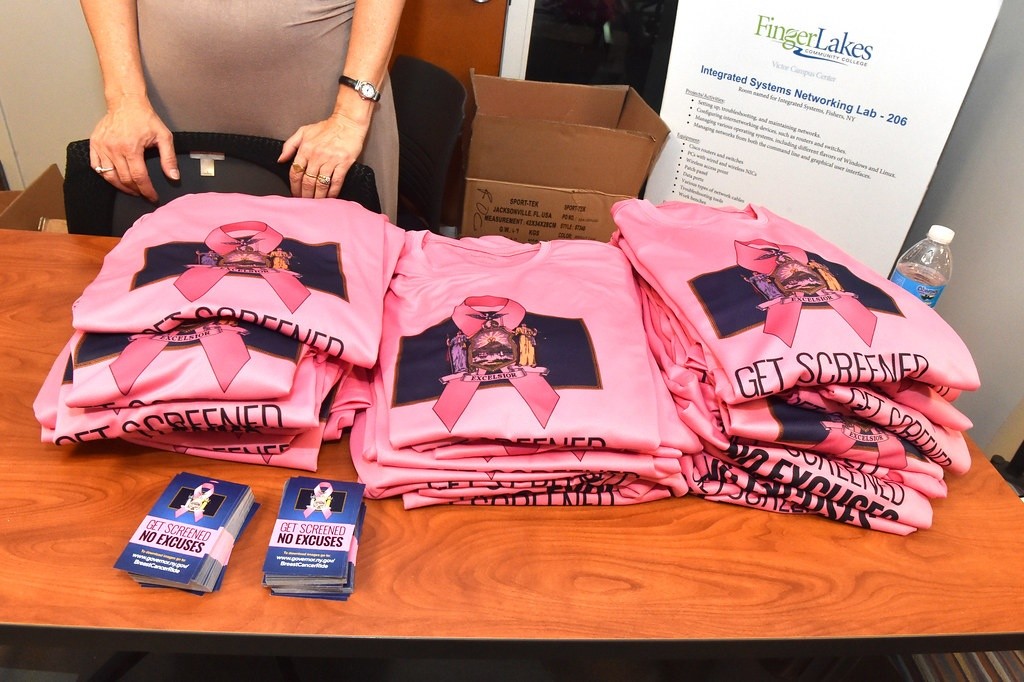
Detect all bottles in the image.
[889,224,954,308]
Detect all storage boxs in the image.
[456,67,670,244]
[0,163,69,232]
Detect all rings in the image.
[317,175,331,185]
[305,172,318,178]
[95,166,114,173]
[292,162,305,173]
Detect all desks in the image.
[0,230,1024,682]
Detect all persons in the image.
[80,0,406,227]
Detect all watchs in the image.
[338,75,382,102]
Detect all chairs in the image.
[390,52,467,232]
